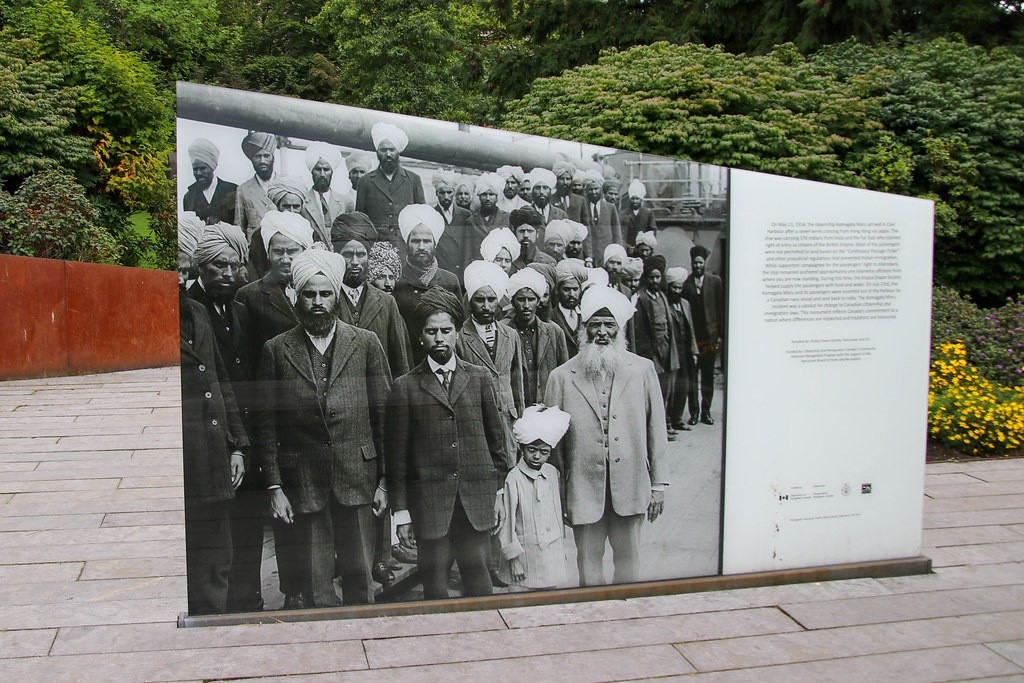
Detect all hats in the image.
[331,211,379,256]
[398,202,445,251]
[367,239,402,284]
[290,246,346,306]
[183,119,457,207]
[192,220,250,267]
[414,284,467,332]
[457,160,710,283]
[260,208,317,254]
[464,259,508,305]
[507,266,547,304]
[581,283,640,330]
[177,210,207,257]
[512,403,572,451]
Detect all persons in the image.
[256,248,395,609]
[504,268,557,408]
[330,212,414,587]
[178,121,664,359]
[497,404,571,592]
[542,283,671,588]
[234,209,317,608]
[188,222,270,614]
[382,285,506,601]
[553,258,589,359]
[662,267,701,435]
[454,260,526,587]
[682,245,725,426]
[628,255,681,442]
[178,208,252,615]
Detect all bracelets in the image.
[232,453,246,458]
[378,485,387,492]
